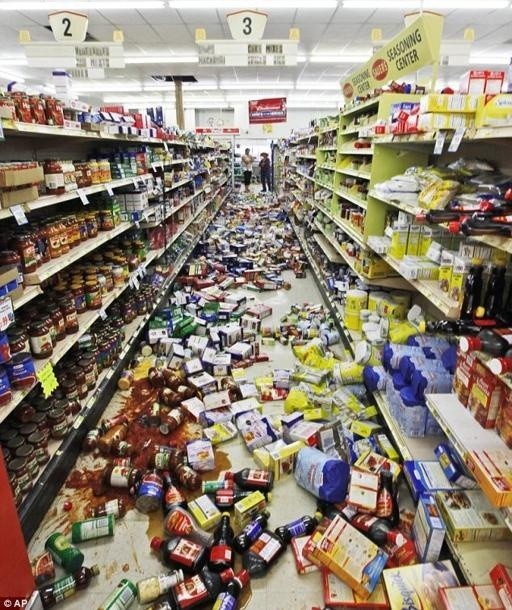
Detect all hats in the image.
[261,153,268,157]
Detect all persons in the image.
[239,148,257,193]
[259,152,273,193]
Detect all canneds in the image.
[0,158,202,610]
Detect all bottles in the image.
[414,182,512,358]
[137,461,399,610]
[39,564,101,610]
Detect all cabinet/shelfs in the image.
[1,120,234,551]
[272,94,512,610]
